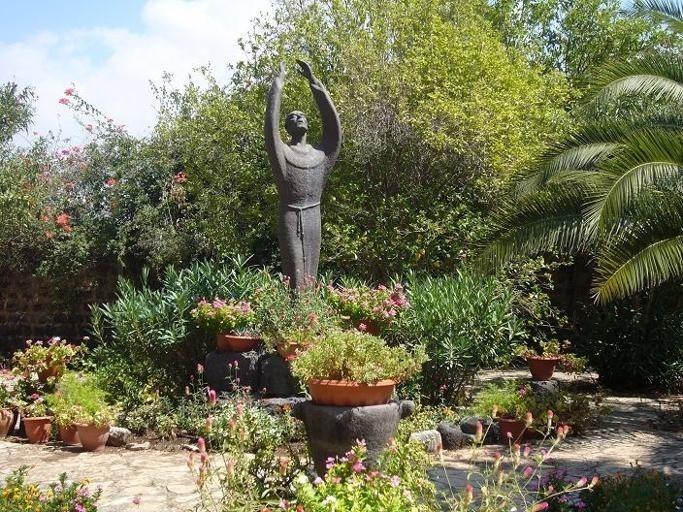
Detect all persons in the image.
[265,54,342,301]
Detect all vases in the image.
[356,320,382,336]
[216,335,259,353]
[34,362,64,385]
[22,417,52,444]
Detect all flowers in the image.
[319,280,411,321]
[12,330,84,365]
[21,394,49,418]
[194,290,251,331]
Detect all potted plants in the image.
[256,282,313,358]
[518,333,562,380]
[50,384,111,451]
[0,382,19,439]
[306,333,406,405]
[494,381,530,444]
